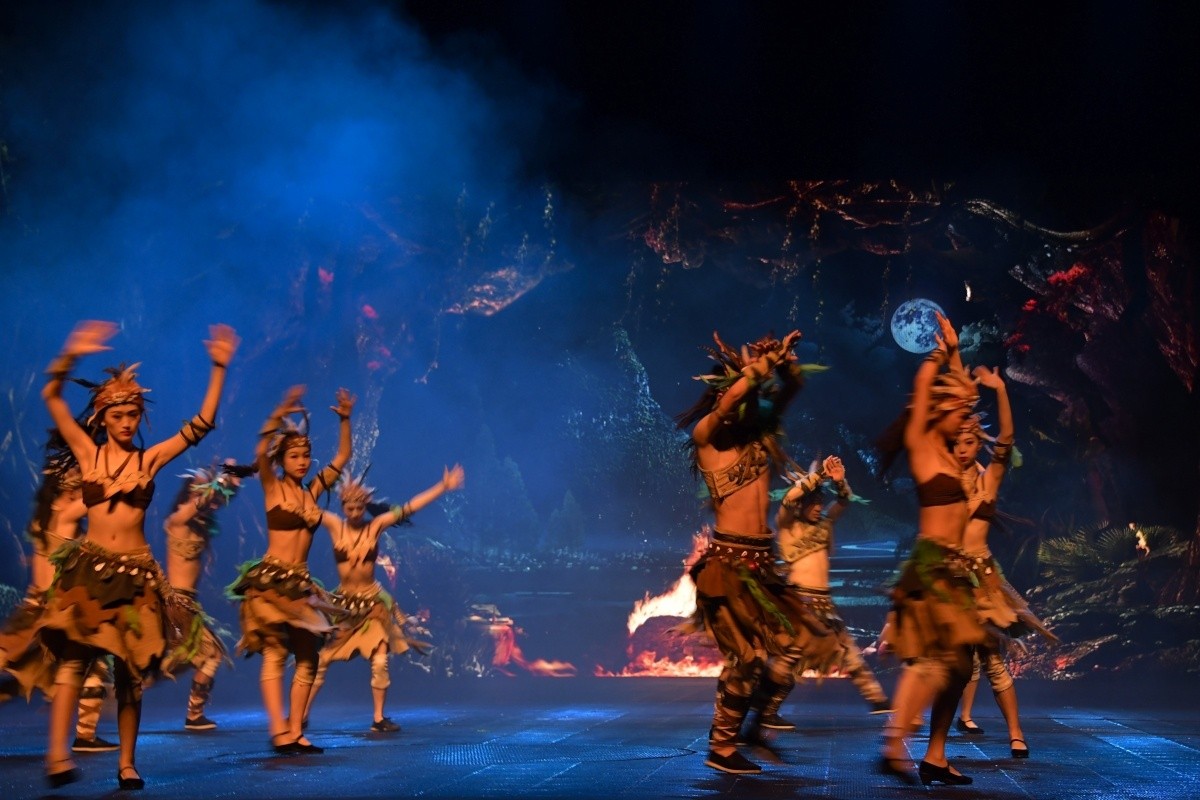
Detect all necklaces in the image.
[225,385,359,756]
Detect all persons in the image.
[912,309,1062,759]
[957,647,986,734]
[287,462,466,732]
[0,450,121,752]
[42,320,242,790]
[690,331,835,774]
[876,332,1024,786]
[759,456,898,730]
[138,457,241,730]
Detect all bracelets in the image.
[924,348,948,367]
[214,362,228,369]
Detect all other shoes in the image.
[46,757,82,786]
[272,731,299,754]
[297,734,324,753]
[1010,739,1028,759]
[958,718,984,733]
[920,762,974,786]
[118,766,144,790]
[877,757,919,785]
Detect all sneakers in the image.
[760,714,797,729]
[184,714,217,729]
[705,750,762,774]
[371,717,400,732]
[73,736,121,751]
[711,723,748,746]
[868,699,900,715]
